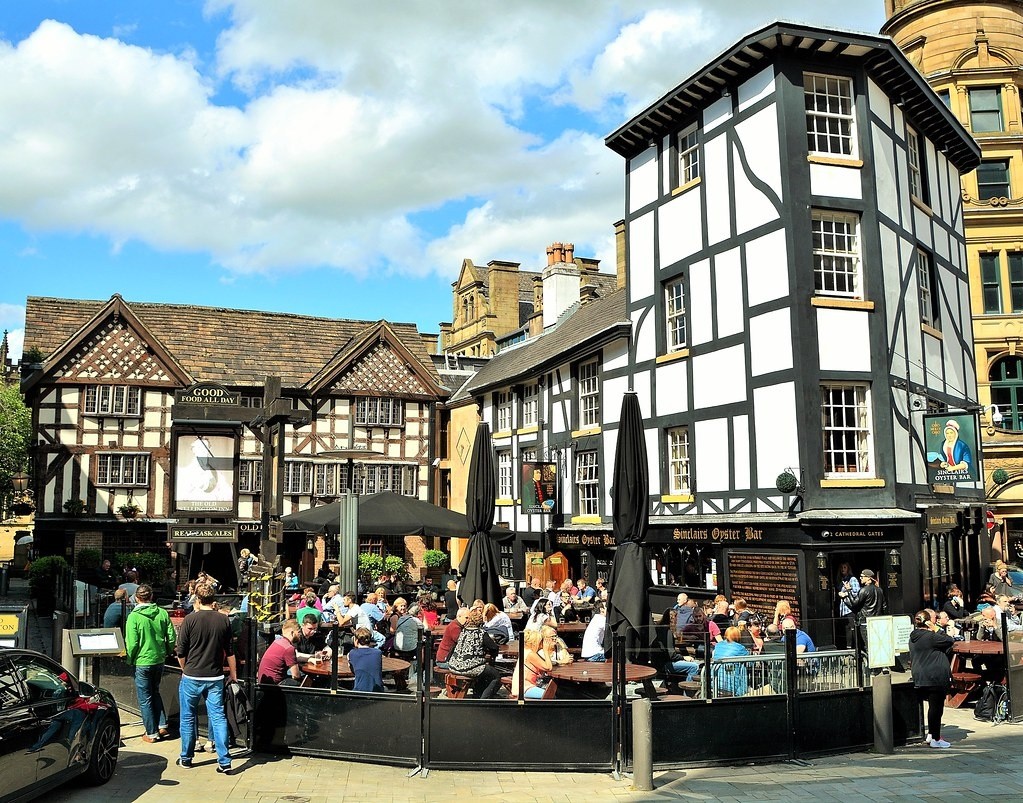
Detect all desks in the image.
[496,643,520,656]
[945,641,1023,709]
[298,655,412,693]
[430,624,449,635]
[505,612,523,619]
[541,662,658,701]
[435,603,448,614]
[555,623,587,653]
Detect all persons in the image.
[161,568,177,601]
[92,559,140,628]
[162,548,223,614]
[125,585,175,743]
[237,549,607,699]
[176,583,237,773]
[835,561,889,676]
[909,558,1023,748]
[651,592,819,696]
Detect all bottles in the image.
[1003,702,1006,715]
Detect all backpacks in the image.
[991,691,1009,728]
[974,685,996,720]
[750,612,771,635]
[997,583,1013,598]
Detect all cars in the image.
[1,651,120,803]
[990,561,1023,608]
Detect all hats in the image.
[862,569,876,583]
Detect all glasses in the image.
[695,614,703,618]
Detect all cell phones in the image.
[925,620,934,628]
[953,596,958,602]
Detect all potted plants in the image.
[116,504,143,519]
[63,498,84,516]
[420,548,448,584]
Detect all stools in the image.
[657,695,692,701]
[948,673,981,708]
[496,659,518,666]
[691,675,701,682]
[434,665,517,699]
[668,674,688,695]
[678,681,702,699]
[635,687,668,697]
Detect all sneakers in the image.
[216,765,231,775]
[929,739,950,748]
[925,734,944,744]
[176,758,192,769]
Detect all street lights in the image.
[252,398,313,624]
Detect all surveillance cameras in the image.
[992,412,1003,424]
[432,457,441,468]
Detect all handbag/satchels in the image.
[488,626,509,646]
[383,637,395,654]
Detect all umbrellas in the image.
[603,388,659,662]
[282,490,516,578]
[456,420,504,612]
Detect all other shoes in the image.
[193,740,202,752]
[202,745,215,754]
[142,734,157,744]
[158,728,169,735]
[401,675,408,680]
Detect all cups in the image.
[315,651,322,666]
[964,631,970,643]
[330,615,334,623]
[338,646,344,657]
[585,617,590,628]
[559,618,565,628]
